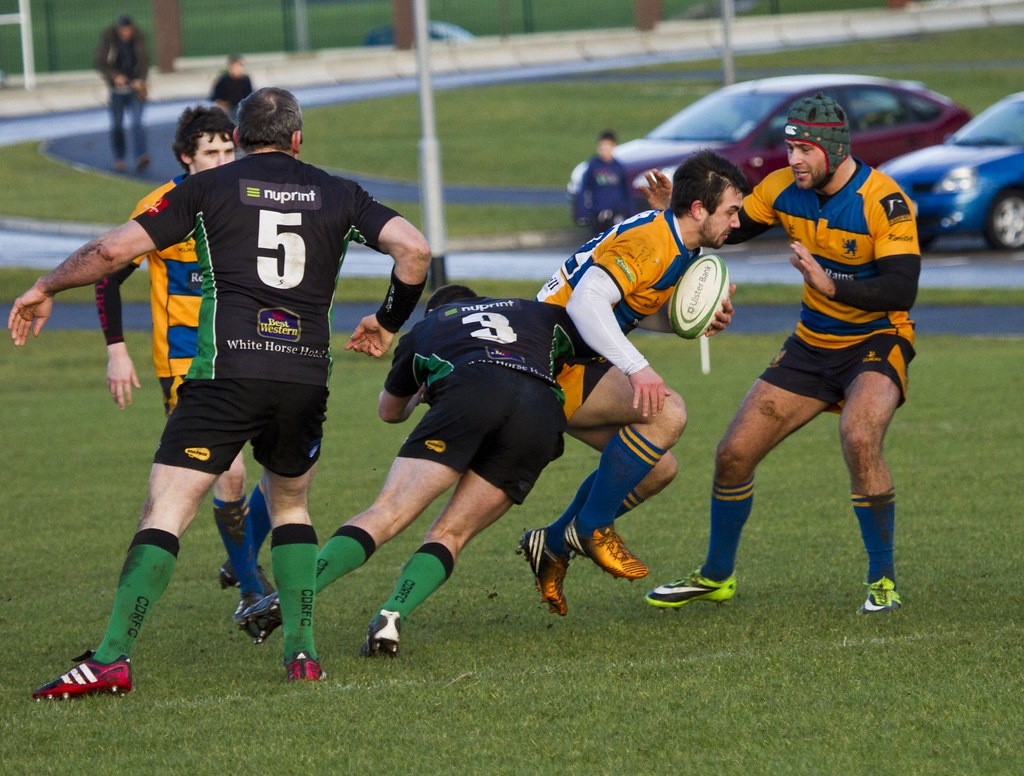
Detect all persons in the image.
[242,285,587,656]
[575,128,632,232]
[8,87,431,701]
[639,93,921,615]
[95,104,318,619]
[96,16,152,172]
[520,151,750,616]
[208,52,253,122]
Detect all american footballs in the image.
[665,254,731,340]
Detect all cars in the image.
[570,71,972,242]
[881,87,1024,255]
[365,20,468,48]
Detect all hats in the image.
[783,92,851,179]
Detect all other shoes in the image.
[135,153,151,171]
[115,159,125,172]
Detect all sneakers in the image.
[219,558,275,597]
[233,591,283,644]
[643,568,737,609]
[518,528,568,617]
[359,609,400,659]
[862,578,902,615]
[283,650,327,684]
[563,515,649,583]
[32,649,132,702]
